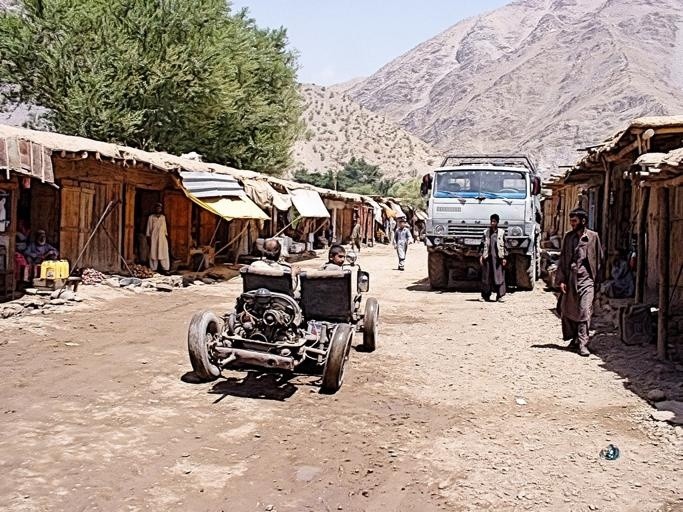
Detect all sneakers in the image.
[566,340,590,355]
[498,298,505,302]
[479,297,486,302]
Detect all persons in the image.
[604,248,634,299]
[251,239,300,290]
[393,220,413,270]
[477,213,507,303]
[554,205,604,357]
[316,244,346,271]
[350,220,360,253]
[22,229,59,265]
[145,202,170,275]
[317,223,330,249]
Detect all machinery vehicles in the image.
[189,251,380,396]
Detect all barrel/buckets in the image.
[40,260,61,279]
[618,299,633,340]
[622,304,652,344]
[60,260,69,279]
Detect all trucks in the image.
[421,154,543,290]
